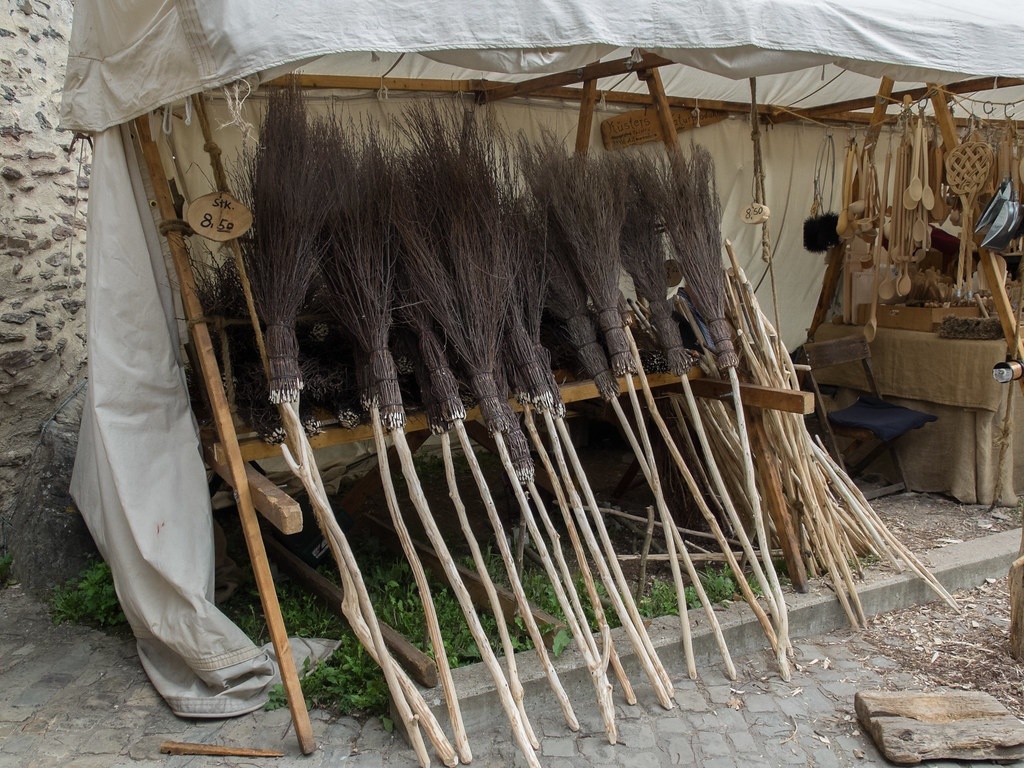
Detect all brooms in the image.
[158,71,794,767]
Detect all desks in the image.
[809,324,1024,507]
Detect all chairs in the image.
[799,333,937,500]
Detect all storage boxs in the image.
[859,302,979,330]
